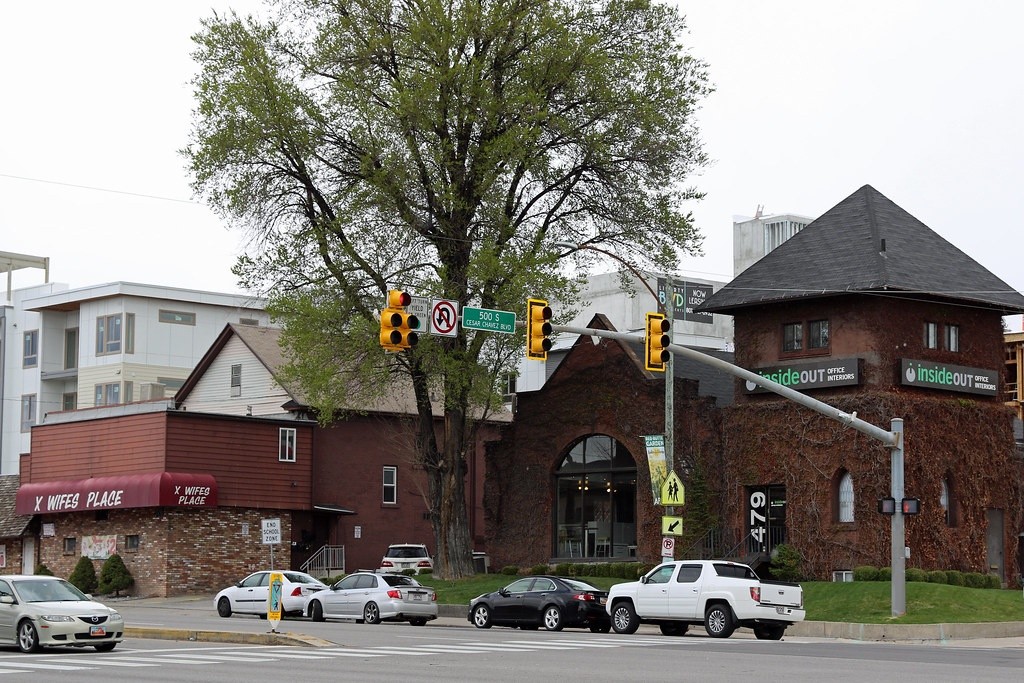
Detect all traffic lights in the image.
[525,299,551,362]
[644,311,671,372]
[878,497,896,516]
[380,289,419,351]
[901,498,920,515]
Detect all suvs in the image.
[378,543,436,575]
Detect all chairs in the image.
[563,529,583,558]
[597,536,610,557]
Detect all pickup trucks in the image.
[605,560,806,640]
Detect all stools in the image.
[627,545,637,557]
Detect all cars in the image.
[302,569,439,627]
[467,575,612,633]
[0,574,124,654]
[213,569,329,620]
